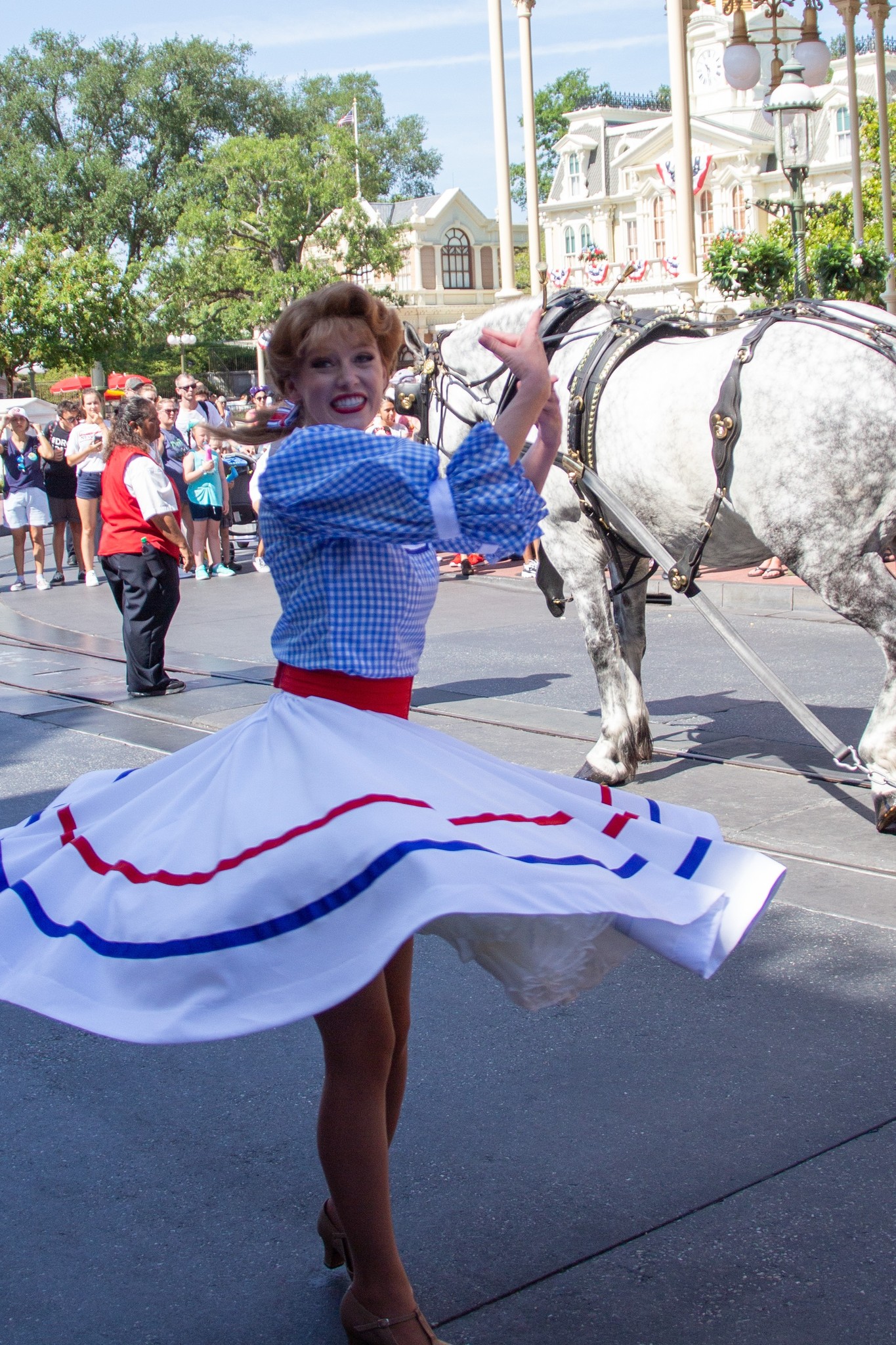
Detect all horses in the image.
[397,287,896,838]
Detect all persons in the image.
[521,537,540,578]
[97,396,186,698]
[66,398,104,565]
[195,379,282,453]
[0,279,789,1345]
[207,436,242,573]
[249,437,287,572]
[41,400,86,585]
[748,556,785,579]
[365,395,409,438]
[172,372,254,560]
[182,418,235,580]
[124,376,209,579]
[64,388,112,586]
[0,407,54,591]
[449,553,484,575]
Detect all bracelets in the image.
[238,445,242,451]
[200,466,206,473]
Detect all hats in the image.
[249,386,264,396]
[8,407,29,421]
[125,377,145,389]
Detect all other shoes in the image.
[78,571,86,581]
[209,563,214,573]
[67,556,78,564]
[252,554,270,573]
[660,569,701,581]
[223,561,242,569]
[50,571,65,585]
[883,553,895,563]
[449,553,467,566]
[10,579,26,591]
[36,578,51,590]
[203,559,209,568]
[468,554,485,566]
[178,567,193,578]
[86,569,100,586]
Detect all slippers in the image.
[762,568,785,579]
[748,565,765,576]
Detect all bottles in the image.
[141,537,152,554]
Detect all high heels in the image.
[316,1199,358,1280]
[341,1285,452,1345]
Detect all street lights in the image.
[766,49,825,298]
[167,324,199,376]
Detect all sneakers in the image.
[195,564,209,580]
[522,559,538,579]
[126,678,185,696]
[211,563,236,577]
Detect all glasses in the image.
[178,383,196,391]
[221,401,226,404]
[148,397,159,402]
[257,395,267,400]
[60,413,80,422]
[16,454,25,471]
[160,408,178,414]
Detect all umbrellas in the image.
[108,373,152,390]
[108,371,122,379]
[50,374,92,394]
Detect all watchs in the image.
[37,433,45,437]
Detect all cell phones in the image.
[94,436,102,446]
